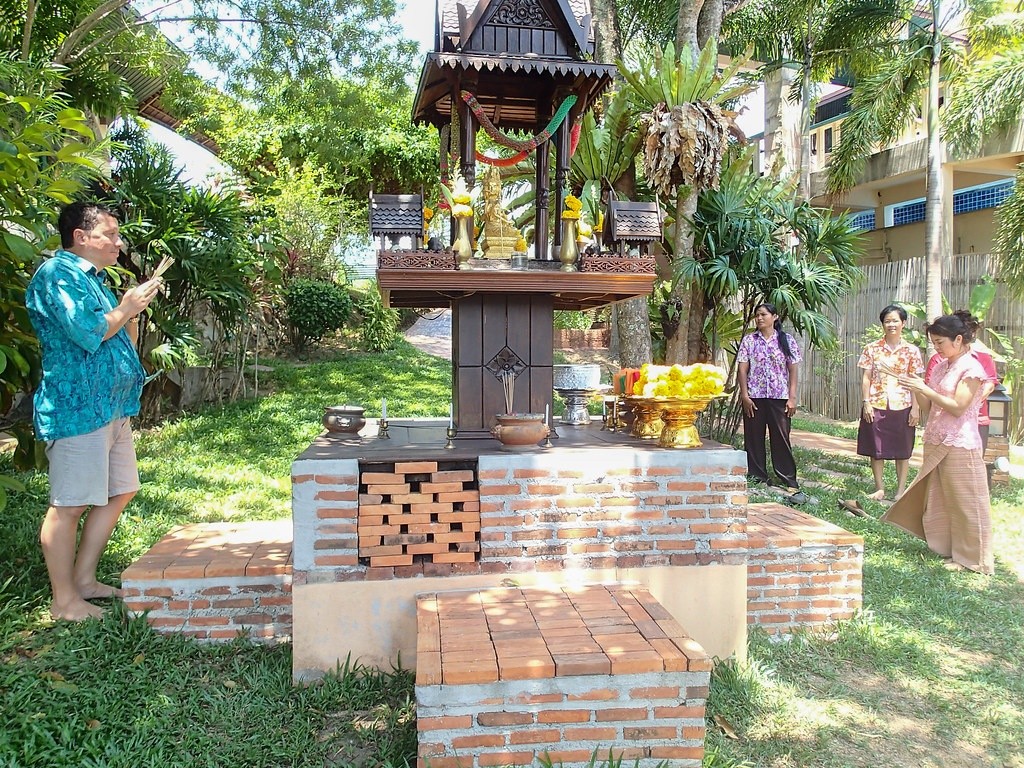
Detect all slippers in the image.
[788,492,818,506]
[837,498,877,520]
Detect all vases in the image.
[452,215,472,270]
[559,218,577,272]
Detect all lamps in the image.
[985,383,1012,437]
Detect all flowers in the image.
[561,194,581,218]
[449,155,473,217]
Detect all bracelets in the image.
[863,399,871,402]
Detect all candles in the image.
[544,404,549,426]
[603,396,605,414]
[613,397,616,418]
[383,396,386,423]
[450,403,453,431]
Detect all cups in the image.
[510,251,528,271]
[552,245,561,262]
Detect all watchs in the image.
[130,314,140,322]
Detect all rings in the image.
[917,423,919,425]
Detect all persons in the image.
[925,341,999,459]
[735,303,803,493]
[879,309,994,576]
[856,304,926,501]
[25,201,159,622]
